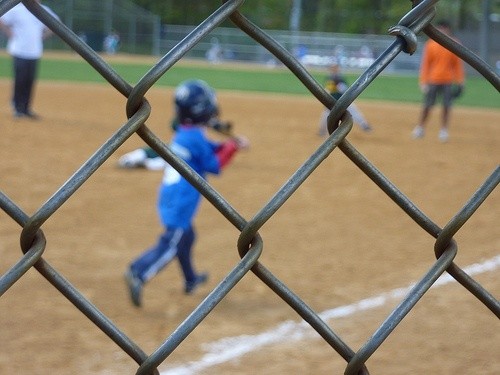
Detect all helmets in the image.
[173,78,220,122]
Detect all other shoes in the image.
[182,271,210,294]
[411,125,424,139]
[125,269,144,306]
[439,129,449,142]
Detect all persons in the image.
[411,19,464,144]
[0,0,64,118]
[119,120,252,175]
[114,79,251,309]
[315,62,372,136]
